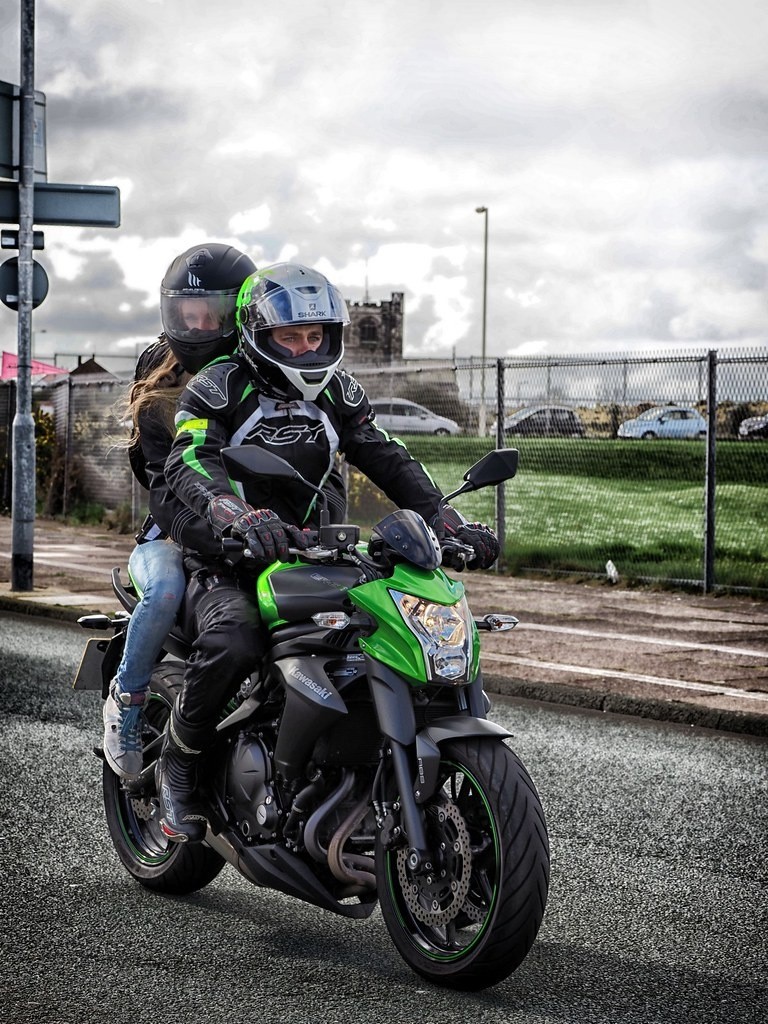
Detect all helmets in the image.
[235,263,352,402]
[160,243,257,377]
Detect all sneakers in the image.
[103,676,151,779]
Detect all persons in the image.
[101,243,259,782]
[153,261,498,846]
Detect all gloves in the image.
[204,494,308,564]
[432,504,501,572]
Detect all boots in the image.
[156,690,218,842]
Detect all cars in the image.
[617,405,708,443]
[368,396,463,438]
[491,404,587,440]
[737,412,768,441]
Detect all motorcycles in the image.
[71,445,551,995]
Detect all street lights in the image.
[476,205,489,439]
